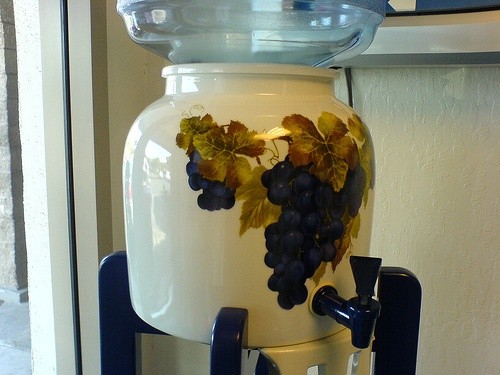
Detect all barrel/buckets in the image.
[115,0,387,66]
